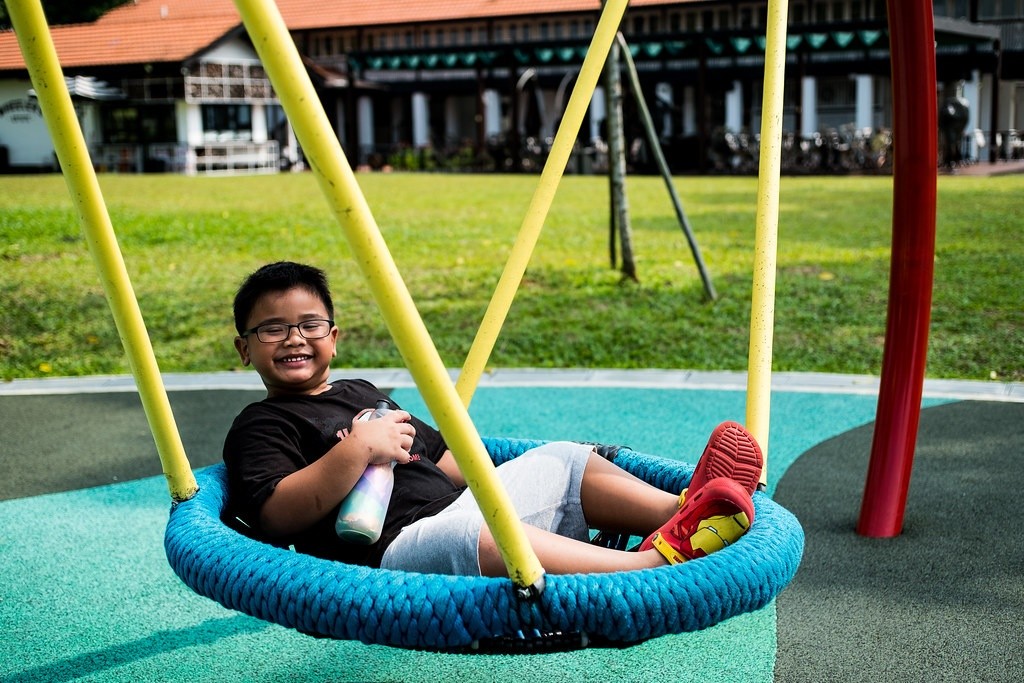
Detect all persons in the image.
[224,263,764,574]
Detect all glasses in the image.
[241,319,335,343]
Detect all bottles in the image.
[335,398,399,547]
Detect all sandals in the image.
[678,422,764,511]
[638,477,755,566]
